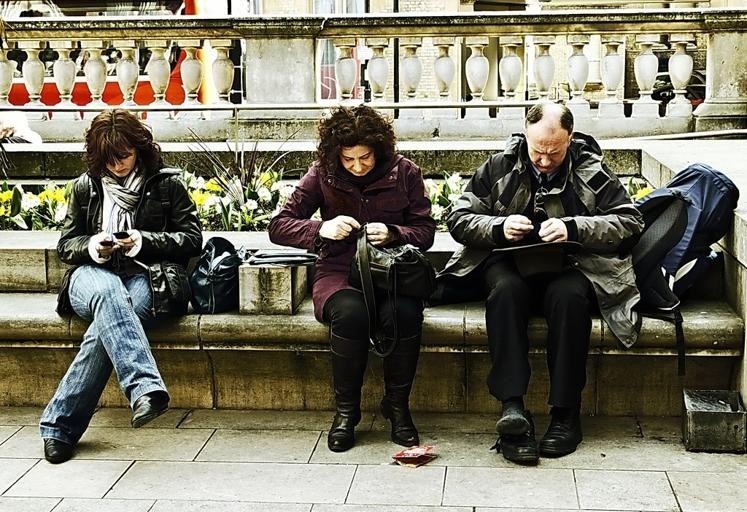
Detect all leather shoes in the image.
[500,411,537,465]
[539,408,582,457]
[44,438,73,464]
[131,390,170,428]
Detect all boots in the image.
[328,333,368,452]
[380,336,420,447]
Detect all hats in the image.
[493,242,584,288]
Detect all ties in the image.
[539,173,549,185]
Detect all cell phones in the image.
[100,241,114,246]
[113,232,129,239]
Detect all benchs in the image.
[0,244,739,420]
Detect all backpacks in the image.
[624,163,738,379]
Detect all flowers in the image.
[423,170,466,229]
[185,164,284,230]
[0,177,70,232]
[622,182,655,204]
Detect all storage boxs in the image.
[683,388,747,455]
[238,250,308,315]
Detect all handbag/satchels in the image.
[188,237,318,314]
[346,222,438,358]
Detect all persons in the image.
[39,106,201,463]
[445,101,644,464]
[267,106,433,453]
